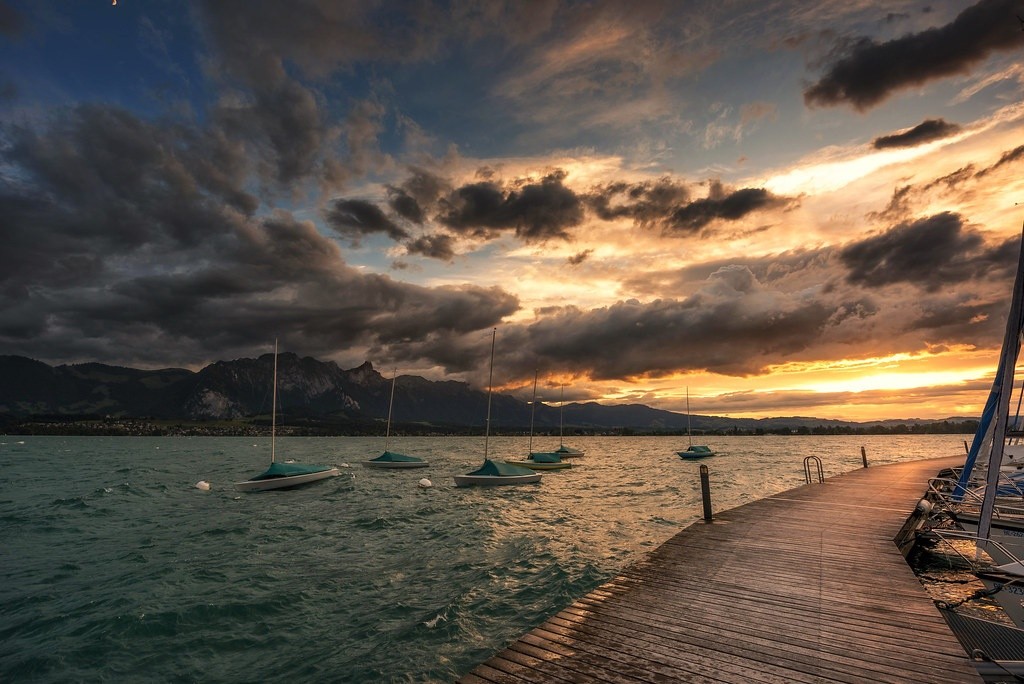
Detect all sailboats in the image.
[503,368,572,471]
[361,368,429,469]
[453,326,543,489]
[233,338,340,493]
[539,383,583,459]
[676,386,717,458]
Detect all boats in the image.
[931,528,1024,631]
[927,476,1024,566]
